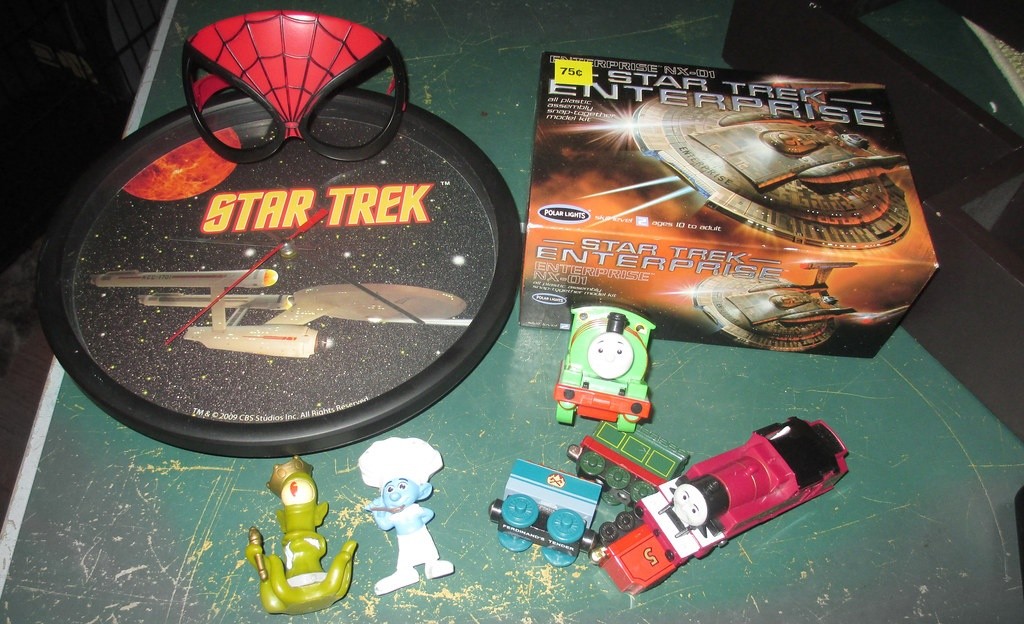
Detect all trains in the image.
[486,305,848,595]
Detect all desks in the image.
[0,0,1024,624]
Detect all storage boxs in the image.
[519,51,939,359]
